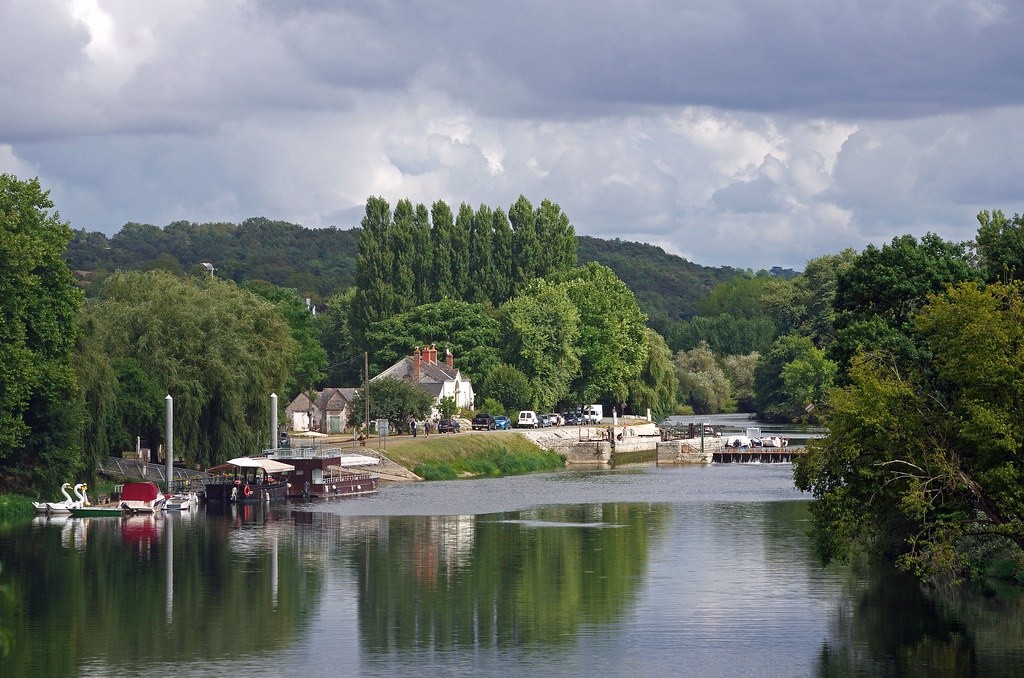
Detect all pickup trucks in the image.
[472,413,496,431]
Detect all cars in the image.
[549,413,566,426]
[494,416,512,430]
[541,415,552,427]
[438,419,460,434]
[281,432,290,447]
[562,413,578,425]
[575,412,587,425]
[535,413,544,428]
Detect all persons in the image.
[556,414,561,428]
[412,419,418,437]
[424,420,431,438]
[359,433,366,446]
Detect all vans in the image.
[517,411,540,429]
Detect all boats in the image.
[205,458,295,502]
[692,417,714,435]
[727,427,788,448]
[30,482,198,518]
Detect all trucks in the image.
[583,404,603,425]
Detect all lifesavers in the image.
[243,485,251,497]
